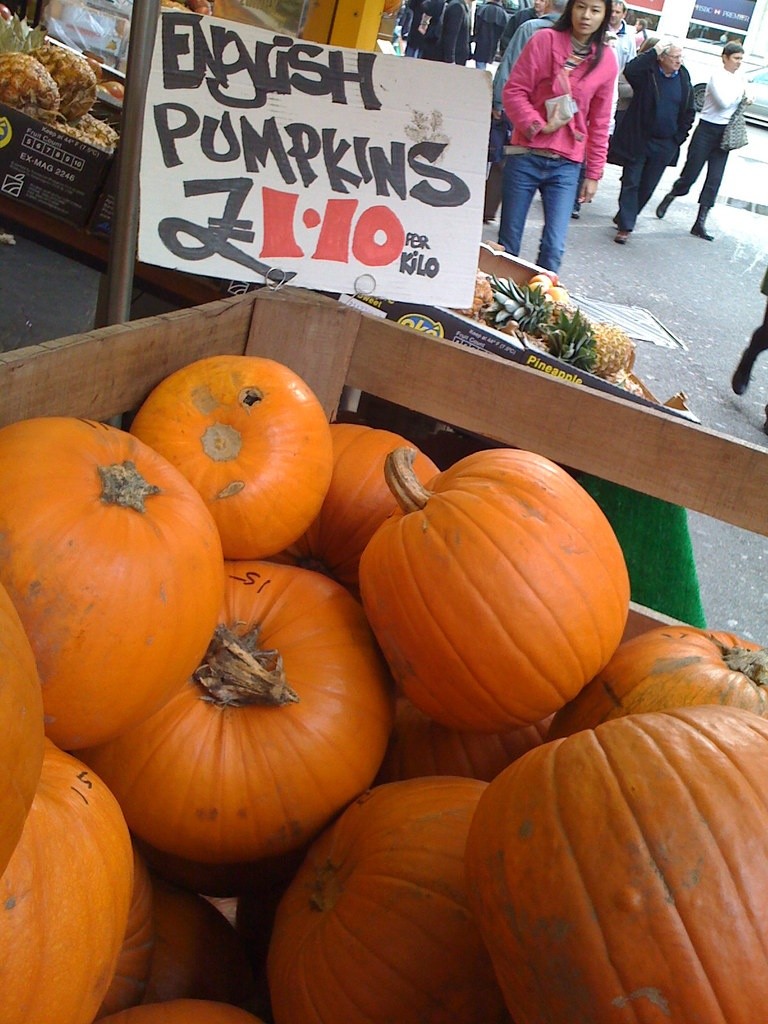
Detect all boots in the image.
[657,194,676,218]
[691,205,714,240]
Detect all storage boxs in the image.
[340,241,703,475]
[89,150,121,238]
[0,20,127,224]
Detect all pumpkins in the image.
[0,356,768,1024]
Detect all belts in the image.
[502,145,561,159]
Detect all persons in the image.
[399,0,754,279]
[731,266,768,434]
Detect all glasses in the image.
[663,51,683,60]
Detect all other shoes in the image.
[732,349,756,395]
[616,230,628,243]
[572,208,579,218]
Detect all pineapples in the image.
[0,11,122,152]
[451,268,646,398]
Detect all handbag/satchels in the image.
[720,102,748,151]
